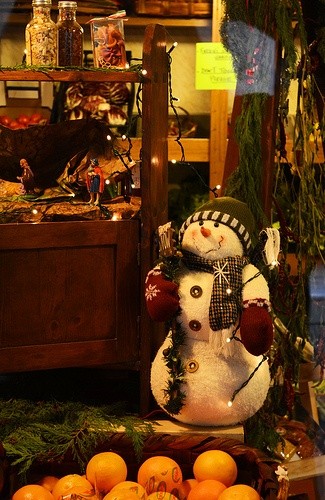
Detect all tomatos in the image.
[0,113,47,130]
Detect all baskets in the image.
[0,432,287,500]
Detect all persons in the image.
[16,158,36,195]
[85,158,106,208]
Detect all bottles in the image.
[56,1,84,66]
[24,0,58,67]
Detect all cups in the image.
[89,19,127,69]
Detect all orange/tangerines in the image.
[10,449,259,500]
